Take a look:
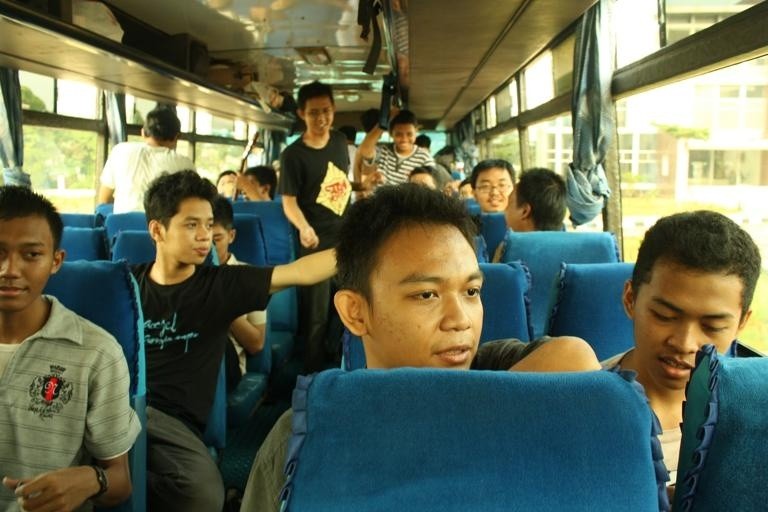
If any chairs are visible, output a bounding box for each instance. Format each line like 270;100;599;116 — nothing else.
39;196;768;512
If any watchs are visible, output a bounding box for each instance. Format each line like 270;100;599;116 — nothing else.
87;462;107;500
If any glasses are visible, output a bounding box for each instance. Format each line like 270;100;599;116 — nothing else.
474;183;513;194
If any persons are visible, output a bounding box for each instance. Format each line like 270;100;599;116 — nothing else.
493;167;568;263
408;134;475;200
130;172;337;511
277;82;384;376
97;110;195;215
2;186;141;511
338;108;382;205
210;195;268;391
471;160;517;227
217;166;277;201
358;96;436;185
603;208;760;499
238;186;600;508
